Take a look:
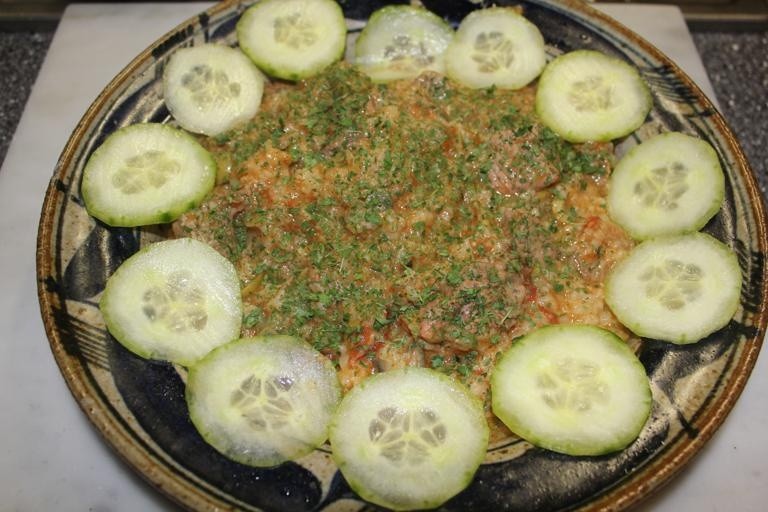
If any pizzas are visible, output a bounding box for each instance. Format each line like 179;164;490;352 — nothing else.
169;59;641;445
